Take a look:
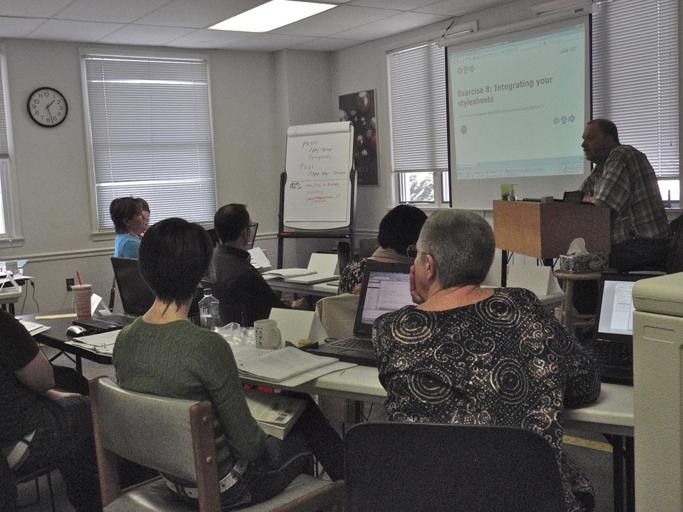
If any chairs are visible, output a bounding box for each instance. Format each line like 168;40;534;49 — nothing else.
110;256;200;321
343;422;592;512
200;279;249;328
1;433;82;511
27;87;69;128
86;375;347;511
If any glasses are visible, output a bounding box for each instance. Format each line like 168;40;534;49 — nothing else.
247;223;257;230
406;244;432;260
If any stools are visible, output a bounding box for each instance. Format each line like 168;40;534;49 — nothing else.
552;269;605;330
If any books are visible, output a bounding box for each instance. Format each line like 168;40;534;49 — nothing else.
17;317;51;338
243;390;305;441
259;267;339;286
229;344;338;382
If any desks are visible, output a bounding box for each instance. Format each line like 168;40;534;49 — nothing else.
479;284;569;315
216;323;634;512
12;308;138;378
492;199;613;289
264;274;343;311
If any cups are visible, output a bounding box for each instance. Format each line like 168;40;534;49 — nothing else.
72;284;92;320
500;183;514;200
254;319;282;350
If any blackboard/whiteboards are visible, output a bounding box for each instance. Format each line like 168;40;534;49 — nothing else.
284;118;354;232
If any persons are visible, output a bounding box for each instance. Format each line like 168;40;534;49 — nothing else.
0;307;104;512
371;208;601;512
338;204;428;298
557;118;669;341
208;203;310;328
109;197;143;260
112;217;346;512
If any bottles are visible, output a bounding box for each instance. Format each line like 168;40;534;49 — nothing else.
197;288;220;330
500;182;517;201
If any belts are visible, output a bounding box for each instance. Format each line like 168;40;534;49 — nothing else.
158;457;250;498
8;427;36;469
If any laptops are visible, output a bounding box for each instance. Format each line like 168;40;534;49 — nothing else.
310;261;416;367
71;313;135;335
580;274;661;387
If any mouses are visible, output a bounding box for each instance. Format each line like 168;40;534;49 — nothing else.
65;325;87;336
